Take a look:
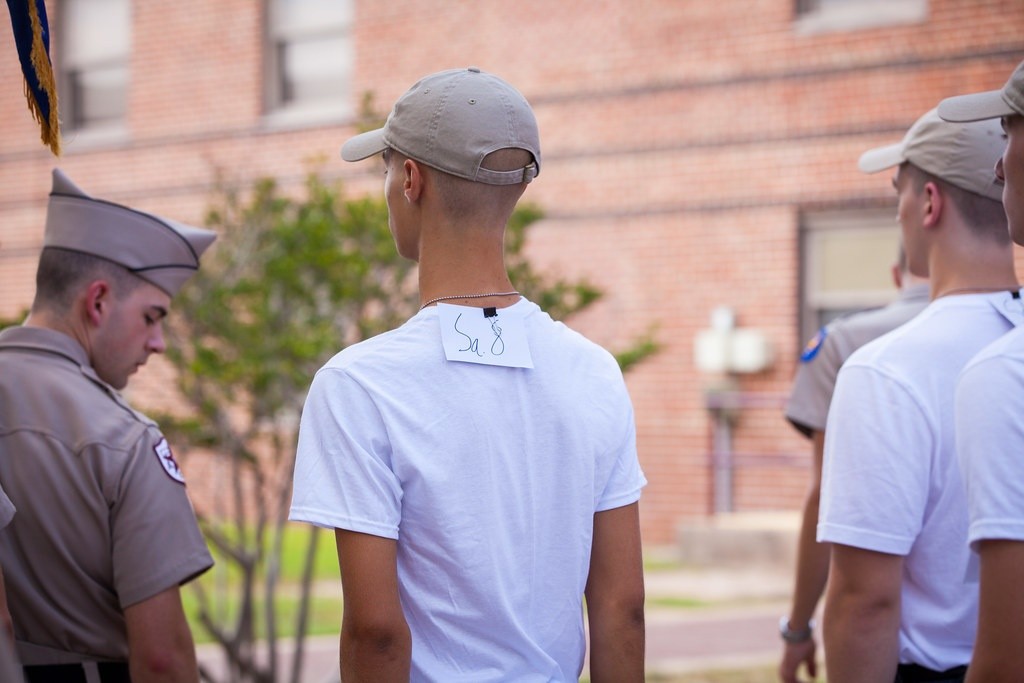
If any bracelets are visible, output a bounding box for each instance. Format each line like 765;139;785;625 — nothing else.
781;621;812;643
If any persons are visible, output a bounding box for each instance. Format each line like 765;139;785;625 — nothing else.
938;60;1024;683
779;241;931;683
816;106;1024;683
0;169;218;683
287;65;650;683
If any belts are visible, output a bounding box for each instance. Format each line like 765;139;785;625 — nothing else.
23;660;131;683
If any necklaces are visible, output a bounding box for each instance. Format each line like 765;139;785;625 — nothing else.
419;291;519;310
930;286;1022;304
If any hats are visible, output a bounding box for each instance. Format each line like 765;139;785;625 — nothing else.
938;63;1024;130
340;67;541;184
42;168;218;301
857;102;1011;203
